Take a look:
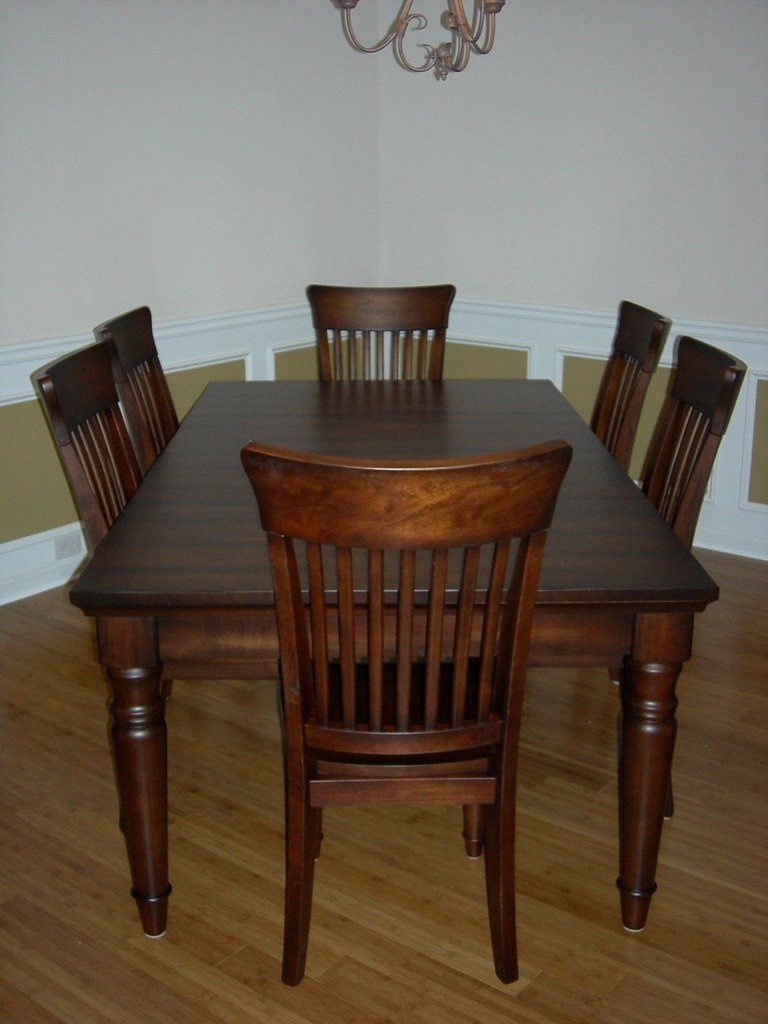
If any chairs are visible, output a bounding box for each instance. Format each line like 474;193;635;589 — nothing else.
588;299;674;472
307;284;457;379
239;439;572;988
93;305;184;477
635;336;749;817
31;339;146;553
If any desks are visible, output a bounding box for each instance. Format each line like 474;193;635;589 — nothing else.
69;380;719;940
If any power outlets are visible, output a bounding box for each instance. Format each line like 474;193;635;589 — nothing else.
55;531;81;560
